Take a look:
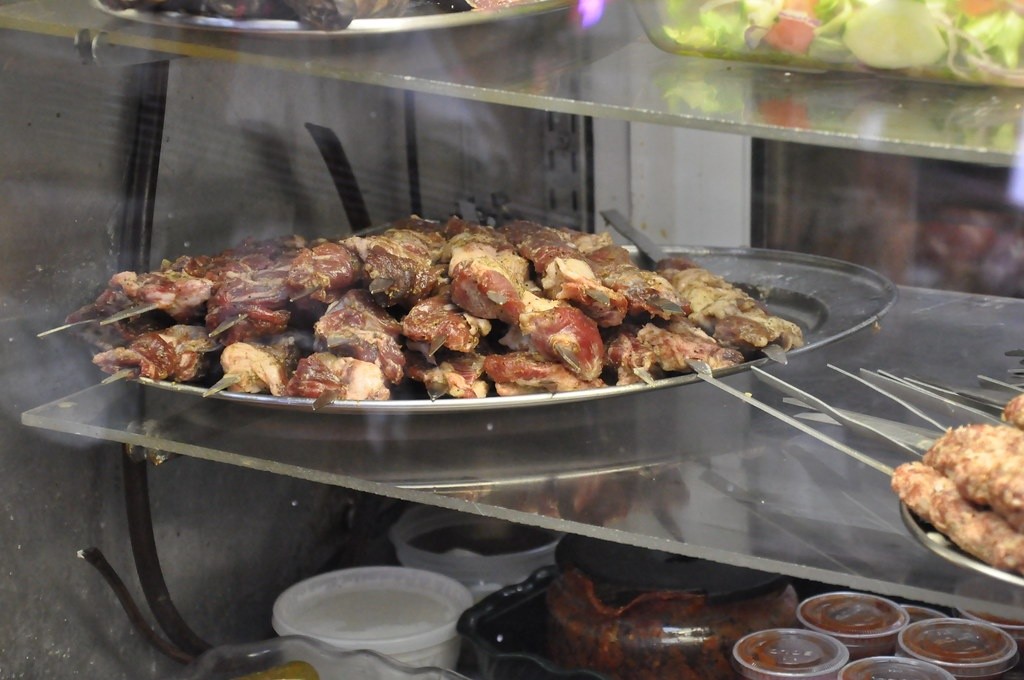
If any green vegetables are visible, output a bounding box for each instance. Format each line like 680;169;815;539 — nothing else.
661;0;1024;75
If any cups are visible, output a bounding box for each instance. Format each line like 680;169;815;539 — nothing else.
269;565;473;680
390;507;562;586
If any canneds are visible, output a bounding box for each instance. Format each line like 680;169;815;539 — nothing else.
731;568;1024;680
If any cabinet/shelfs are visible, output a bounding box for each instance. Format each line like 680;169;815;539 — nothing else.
0;6;1024;680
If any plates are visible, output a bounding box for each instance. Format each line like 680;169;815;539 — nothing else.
64;244;901;417
175;634;477;680
900;500;1024;588
93;0;600;43
